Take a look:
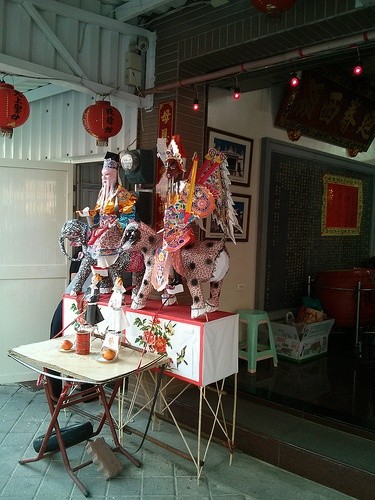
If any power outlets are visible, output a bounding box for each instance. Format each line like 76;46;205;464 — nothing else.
237;282;246;291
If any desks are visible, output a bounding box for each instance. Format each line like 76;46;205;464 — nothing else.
7;331;171;498
59;292;240;488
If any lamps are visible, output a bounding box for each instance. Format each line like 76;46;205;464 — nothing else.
192;85;199;110
352;46;363;75
288;61;299;88
233;76;240;100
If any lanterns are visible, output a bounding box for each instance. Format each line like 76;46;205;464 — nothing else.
0;80;30;139
82;101;123;147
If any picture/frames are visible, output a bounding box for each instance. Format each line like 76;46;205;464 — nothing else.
205;126;254;188
201;192;252;242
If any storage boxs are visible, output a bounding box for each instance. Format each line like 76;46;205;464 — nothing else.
269;318;336;361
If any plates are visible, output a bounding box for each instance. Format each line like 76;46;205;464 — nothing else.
94;355;117;362
56;345;75;352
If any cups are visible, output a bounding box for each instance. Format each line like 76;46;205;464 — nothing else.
76;331;90;354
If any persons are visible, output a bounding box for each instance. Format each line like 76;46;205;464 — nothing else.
83;273;105;325
163;139;243;294
70;247;83;282
83;151;138;277
105;277;131;332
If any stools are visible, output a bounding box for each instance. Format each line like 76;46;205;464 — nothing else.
234;308;278;373
